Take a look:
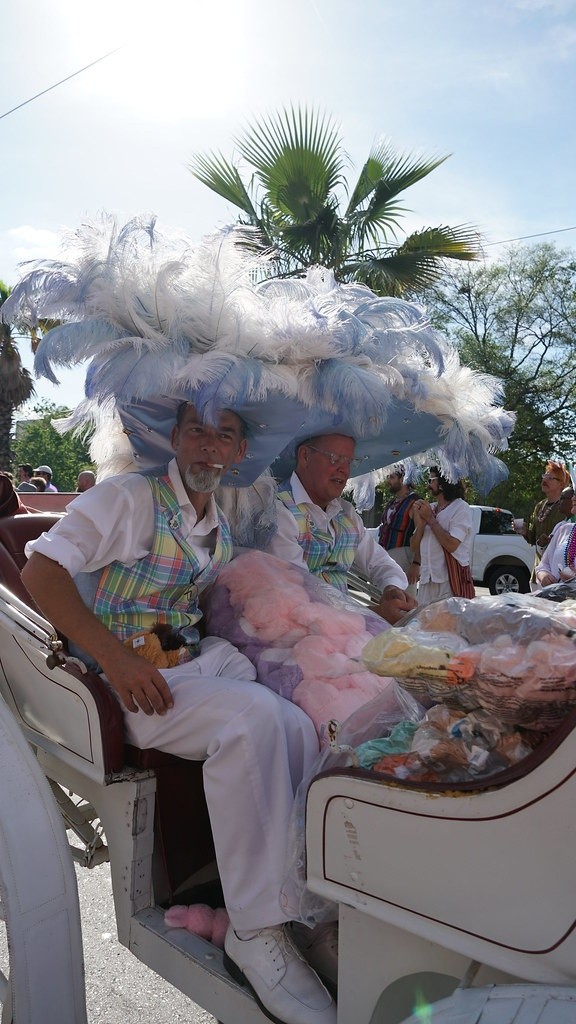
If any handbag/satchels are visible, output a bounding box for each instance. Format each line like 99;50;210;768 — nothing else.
444;549;475;598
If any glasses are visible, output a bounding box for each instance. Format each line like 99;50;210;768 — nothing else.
428;477;440;483
542;475;561;483
307;445;361;470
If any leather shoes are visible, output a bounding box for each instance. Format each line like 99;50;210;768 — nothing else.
222;923;337;1024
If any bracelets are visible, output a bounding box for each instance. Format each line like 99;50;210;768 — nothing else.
413;561;421;566
427;515;439;529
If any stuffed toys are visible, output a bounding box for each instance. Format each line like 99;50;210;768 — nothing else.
204;550;576;784
123;623;200;668
164;904;227;946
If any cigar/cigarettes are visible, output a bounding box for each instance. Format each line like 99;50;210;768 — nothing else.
208;463;225;468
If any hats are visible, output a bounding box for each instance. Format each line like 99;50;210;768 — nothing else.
15;482;37;492
33;465;52;476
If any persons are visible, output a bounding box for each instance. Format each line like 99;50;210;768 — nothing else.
536;487;574;556
17;464;34;483
410;466;474;605
535;492;576;587
78;471;96;492
19;402;339;1024
32;466;57;492
29;477;46;492
266;433;420;626
512;459;571;593
378;464;425;598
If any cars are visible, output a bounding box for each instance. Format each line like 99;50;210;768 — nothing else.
365;503;538;597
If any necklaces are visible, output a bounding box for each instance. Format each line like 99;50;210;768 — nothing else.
438;503;449;512
564;523;576;574
538;498;558;523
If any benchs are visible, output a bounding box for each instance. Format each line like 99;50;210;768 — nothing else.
1;513;225;912
306;717;576;1024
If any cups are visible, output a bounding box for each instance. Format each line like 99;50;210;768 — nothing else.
513;518;524;533
